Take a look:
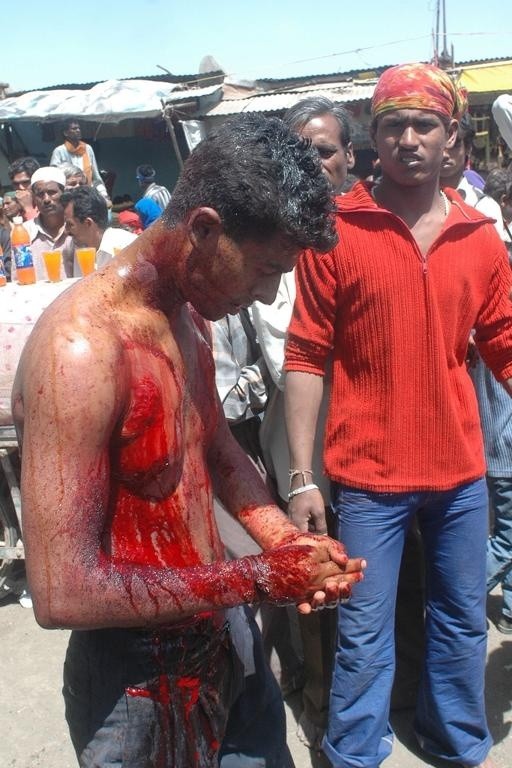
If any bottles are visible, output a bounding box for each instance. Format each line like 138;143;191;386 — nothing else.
11;216;37;285
0;242;7;287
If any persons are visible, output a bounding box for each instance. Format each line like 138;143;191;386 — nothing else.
0;117;172;282
204;62;512;768
12;113;366;768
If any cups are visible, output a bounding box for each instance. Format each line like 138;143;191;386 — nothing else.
44;251;61;283
76;247;96;278
